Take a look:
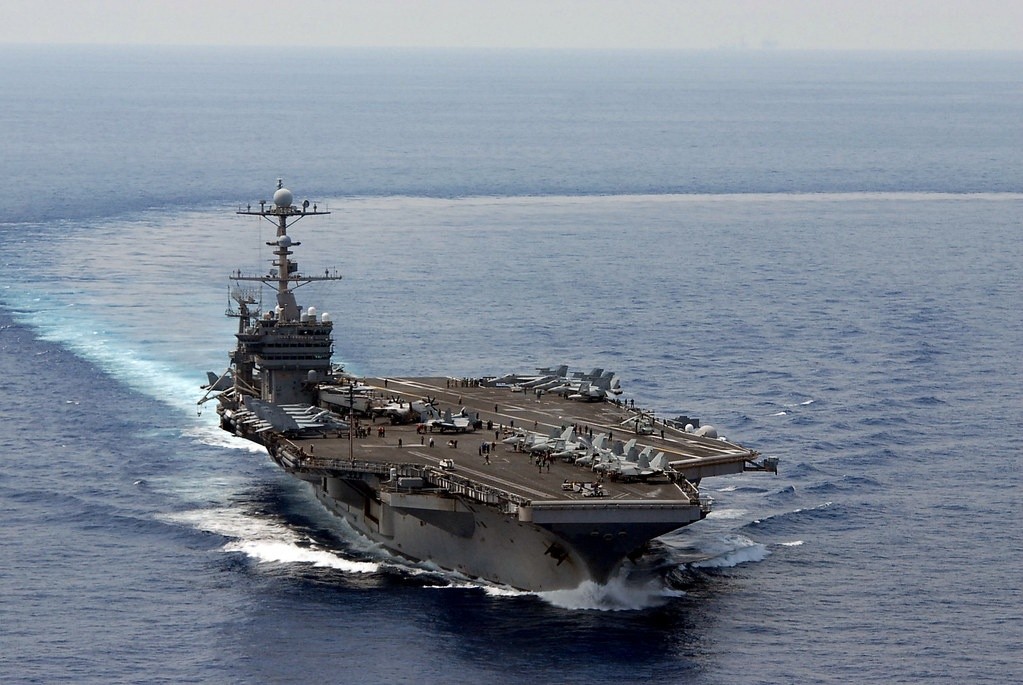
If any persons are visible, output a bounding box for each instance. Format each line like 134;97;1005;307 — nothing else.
520;382;573;400
510;419;555;473
310;444;313;453
339;409;387;441
561;466;621;494
557;419;617;441
639;412;667;440
445;377;482;388
397;396;509;468
384;379;389;388
610;397;637;409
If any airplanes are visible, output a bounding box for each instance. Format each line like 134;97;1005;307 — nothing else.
486;363;624;404
425;406;482;433
500;425;670;482
232;395;351;440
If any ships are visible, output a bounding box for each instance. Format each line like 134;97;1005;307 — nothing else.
196;175;781;608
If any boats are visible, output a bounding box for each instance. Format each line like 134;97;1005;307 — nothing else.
315;378;375;415
